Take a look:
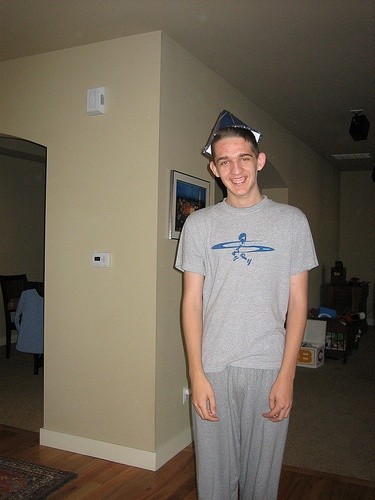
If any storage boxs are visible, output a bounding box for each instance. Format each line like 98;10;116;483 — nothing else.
293;365;309;391
296;319;328;368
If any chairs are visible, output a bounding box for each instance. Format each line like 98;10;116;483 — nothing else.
0;274;45;376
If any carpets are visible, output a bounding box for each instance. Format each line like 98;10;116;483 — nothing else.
0;455;79;500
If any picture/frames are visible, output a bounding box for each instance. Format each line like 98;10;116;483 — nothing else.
169;169;210;240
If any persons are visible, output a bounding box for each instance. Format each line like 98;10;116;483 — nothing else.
174;127;319;500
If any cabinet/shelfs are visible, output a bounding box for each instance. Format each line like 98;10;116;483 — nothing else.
320;284;369;316
